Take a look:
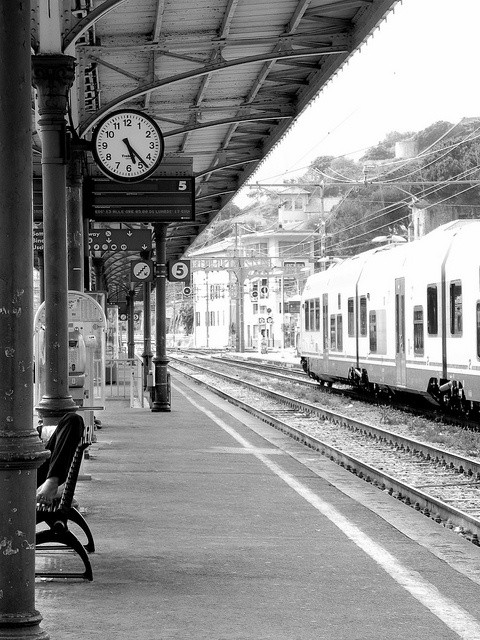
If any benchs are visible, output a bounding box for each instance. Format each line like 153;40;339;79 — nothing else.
35;425;95;581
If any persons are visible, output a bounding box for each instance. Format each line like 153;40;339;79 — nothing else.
36;412;84;505
94;416;102;431
36;420;43;438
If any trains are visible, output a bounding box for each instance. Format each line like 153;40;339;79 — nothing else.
300;219;480;427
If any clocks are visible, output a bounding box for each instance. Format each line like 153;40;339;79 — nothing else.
90;108;165;185
